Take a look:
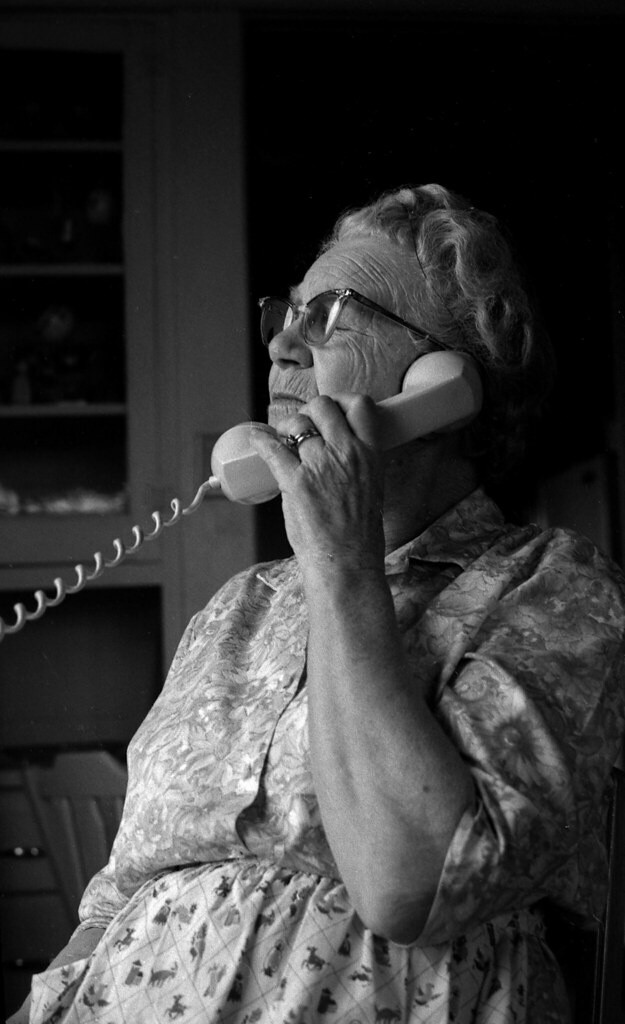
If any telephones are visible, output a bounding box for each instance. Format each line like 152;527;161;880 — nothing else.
211;349;483;509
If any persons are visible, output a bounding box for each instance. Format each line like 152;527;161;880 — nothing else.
0;181;625;1024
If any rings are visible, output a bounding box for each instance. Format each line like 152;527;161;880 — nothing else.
279;427;318;453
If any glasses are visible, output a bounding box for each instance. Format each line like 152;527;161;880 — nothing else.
258;289;448;351
